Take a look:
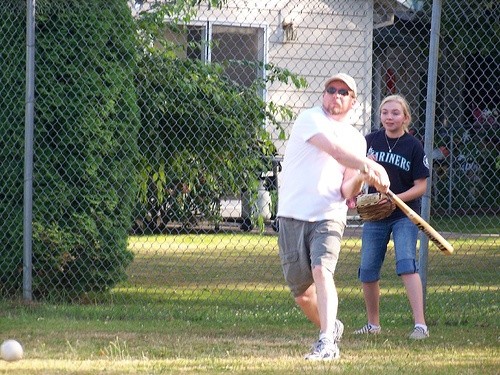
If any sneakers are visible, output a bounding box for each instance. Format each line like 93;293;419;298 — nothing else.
408;327;430;341
303;333;340;362
349;323;382;335
334;319;345;343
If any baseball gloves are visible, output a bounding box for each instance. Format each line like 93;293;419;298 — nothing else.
355;191;397;222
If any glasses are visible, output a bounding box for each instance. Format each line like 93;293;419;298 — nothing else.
326;87;350;96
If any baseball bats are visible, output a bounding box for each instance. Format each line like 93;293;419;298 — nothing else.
359;166;455;257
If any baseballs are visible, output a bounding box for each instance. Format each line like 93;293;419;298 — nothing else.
1;339;25;363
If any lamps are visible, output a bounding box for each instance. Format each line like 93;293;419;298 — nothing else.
281;16;298;42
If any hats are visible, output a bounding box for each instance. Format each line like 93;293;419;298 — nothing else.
325;72;357;98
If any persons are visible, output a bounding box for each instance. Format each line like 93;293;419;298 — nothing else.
433;145;448;159
350;94;431;341
277;73;391;359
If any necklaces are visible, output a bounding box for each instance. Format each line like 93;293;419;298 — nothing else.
384;132;400;152
